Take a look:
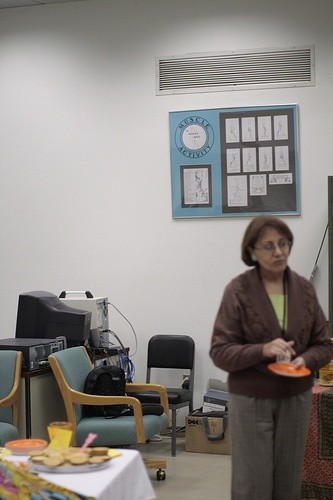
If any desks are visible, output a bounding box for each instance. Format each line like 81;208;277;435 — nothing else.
20;347;130;439
2;447;156;500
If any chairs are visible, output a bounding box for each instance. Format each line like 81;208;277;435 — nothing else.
0;350;22;448
127;334;195;457
48;345;169;481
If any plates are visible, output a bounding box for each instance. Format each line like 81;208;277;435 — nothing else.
268;363;311;378
27;456;113;473
5;439;46;455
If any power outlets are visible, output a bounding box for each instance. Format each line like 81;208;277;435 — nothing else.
183;375;190;382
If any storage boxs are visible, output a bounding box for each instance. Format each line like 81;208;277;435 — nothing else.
202;378;228;413
185;416;231;455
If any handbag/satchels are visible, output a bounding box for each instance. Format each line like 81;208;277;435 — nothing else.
83;365;164;419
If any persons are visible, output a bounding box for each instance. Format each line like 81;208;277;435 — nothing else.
209;217;333;500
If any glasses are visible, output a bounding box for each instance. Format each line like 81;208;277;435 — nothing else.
254;240;291;252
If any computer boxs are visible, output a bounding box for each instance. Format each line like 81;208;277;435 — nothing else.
0;338;65;371
58;297;110;349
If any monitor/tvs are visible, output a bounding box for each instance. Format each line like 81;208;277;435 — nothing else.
15;291;92;349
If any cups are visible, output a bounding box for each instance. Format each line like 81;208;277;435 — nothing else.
49;422;73;448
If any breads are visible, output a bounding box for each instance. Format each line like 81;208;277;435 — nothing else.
29;447;110;466
